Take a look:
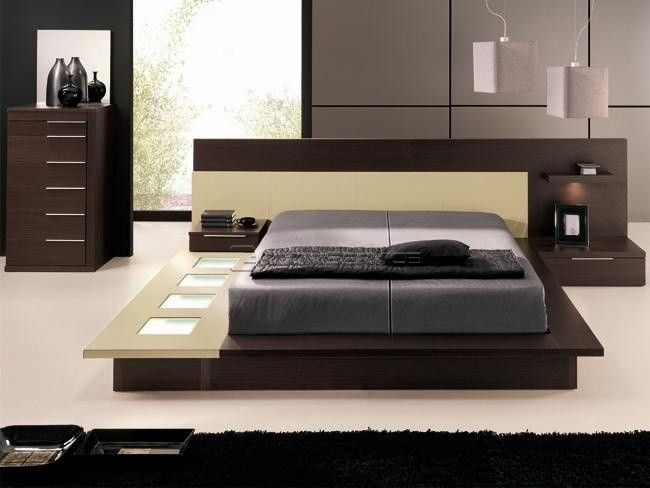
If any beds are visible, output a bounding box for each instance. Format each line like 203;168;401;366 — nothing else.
210;206;605;363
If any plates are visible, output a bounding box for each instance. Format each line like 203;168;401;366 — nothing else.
237;222;258;227
75;427;197;465
0;423;85;475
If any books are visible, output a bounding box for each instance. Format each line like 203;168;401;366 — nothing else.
200;210;236;227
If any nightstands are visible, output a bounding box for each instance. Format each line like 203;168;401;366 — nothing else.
188;218;271;252
532;235;646;287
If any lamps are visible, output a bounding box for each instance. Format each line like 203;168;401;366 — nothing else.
544;0;610;121
469;0;537;94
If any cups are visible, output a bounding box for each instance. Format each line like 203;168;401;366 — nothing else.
236;217;256;225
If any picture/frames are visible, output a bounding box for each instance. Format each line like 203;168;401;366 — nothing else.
553;201;591;248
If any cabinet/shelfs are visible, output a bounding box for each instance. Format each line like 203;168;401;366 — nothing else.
4;104;113;272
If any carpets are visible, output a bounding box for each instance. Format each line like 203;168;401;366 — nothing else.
0;425;648;485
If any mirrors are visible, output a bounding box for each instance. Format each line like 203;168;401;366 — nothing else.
37;28;111;105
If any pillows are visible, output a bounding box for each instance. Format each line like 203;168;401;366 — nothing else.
380;237;473;266
